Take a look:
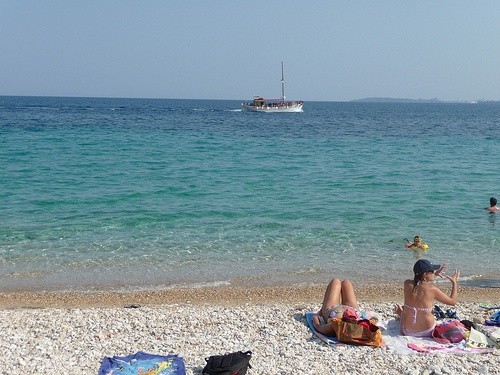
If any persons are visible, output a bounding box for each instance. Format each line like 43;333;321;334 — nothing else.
407;235;430;250
401;258;460;336
484;196;500;212
312;279;380;342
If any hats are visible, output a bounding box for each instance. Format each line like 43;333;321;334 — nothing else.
343;309;359;322
413;259;441;273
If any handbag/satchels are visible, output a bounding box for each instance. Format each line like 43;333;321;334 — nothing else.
436;320;465;343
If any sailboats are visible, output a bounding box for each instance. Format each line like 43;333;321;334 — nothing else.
241;61;305;113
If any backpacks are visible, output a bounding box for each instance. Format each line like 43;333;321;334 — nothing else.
202;350;252;375
